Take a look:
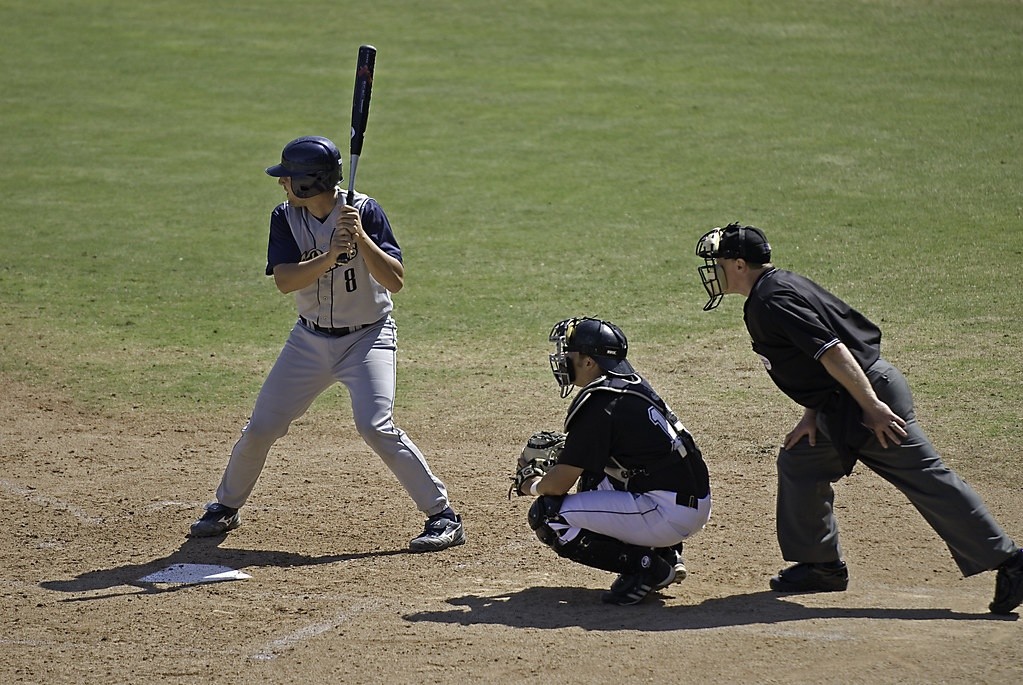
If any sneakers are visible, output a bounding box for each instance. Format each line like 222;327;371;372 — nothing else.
409;514;466;551
769;559;849;593
989;548;1023;613
665;550;687;582
602;554;675;607
189;500;240;536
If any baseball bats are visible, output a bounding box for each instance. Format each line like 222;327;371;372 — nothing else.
335;44;377;265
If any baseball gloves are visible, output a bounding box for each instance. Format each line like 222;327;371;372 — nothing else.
507;429;569;501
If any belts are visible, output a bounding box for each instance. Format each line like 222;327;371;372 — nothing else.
299;316;369;337
675;493;699;510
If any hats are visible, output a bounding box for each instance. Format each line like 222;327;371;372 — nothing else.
698;221;773;261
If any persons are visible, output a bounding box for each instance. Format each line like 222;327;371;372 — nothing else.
189;135;466;551
696;223;1023;615
516;315;711;607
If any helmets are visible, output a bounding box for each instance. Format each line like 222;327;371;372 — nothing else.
558;317;636;376
265;135;344;199
